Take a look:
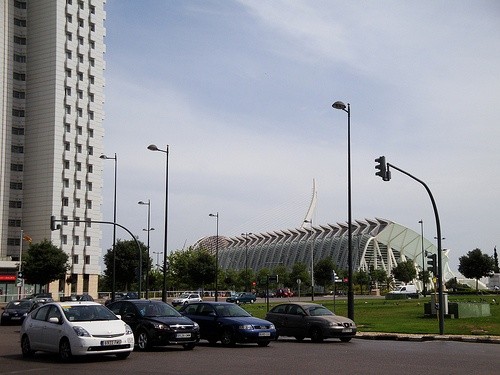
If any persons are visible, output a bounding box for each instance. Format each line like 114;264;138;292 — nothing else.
287;286;291;297
251;288;256;295
279;288;284;298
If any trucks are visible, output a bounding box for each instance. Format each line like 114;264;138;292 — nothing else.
389;285;416;294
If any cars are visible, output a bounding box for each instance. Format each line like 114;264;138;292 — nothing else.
227;292;257;305
102;301;200;353
260;303;357;342
177;302;279;345
0;290;138;325
21;301;136;361
171;292;201;307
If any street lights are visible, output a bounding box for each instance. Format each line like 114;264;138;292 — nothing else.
153;251;164;302
139;200;151;300
146;141;169;303
143;226;156;301
99;152;117;300
419;219;428;297
208;212;219;301
330;100;354;331
241;232;252;292
304;218;315;301
434;237;447;292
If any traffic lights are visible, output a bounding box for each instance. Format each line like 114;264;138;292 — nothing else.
375;155;386;179
427;253;436;275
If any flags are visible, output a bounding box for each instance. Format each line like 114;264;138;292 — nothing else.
22;233;32;243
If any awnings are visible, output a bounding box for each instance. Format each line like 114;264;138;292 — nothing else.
0;274;16;280
